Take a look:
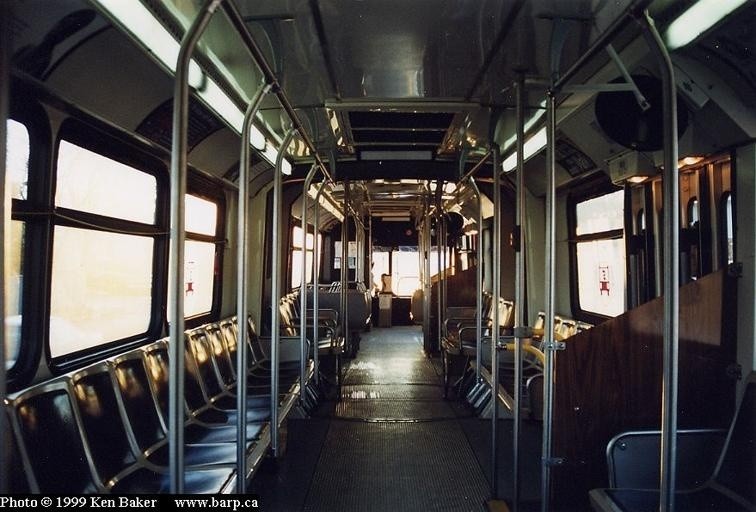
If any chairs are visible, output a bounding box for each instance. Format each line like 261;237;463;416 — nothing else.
588;370;755;512
440;290;595;416
